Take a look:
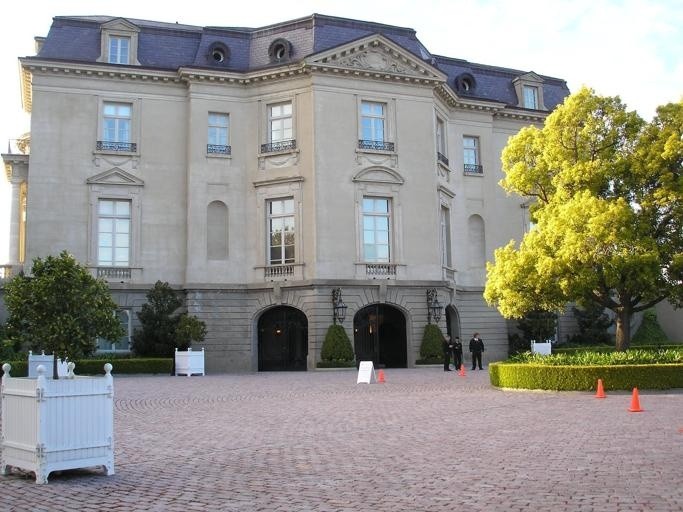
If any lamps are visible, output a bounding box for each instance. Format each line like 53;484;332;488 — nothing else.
331;287;348;324
426;287;443;324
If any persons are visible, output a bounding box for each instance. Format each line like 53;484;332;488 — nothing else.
442;335;454;371
452;336;463;371
469;333;484;370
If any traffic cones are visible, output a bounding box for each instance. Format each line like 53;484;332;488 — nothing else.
379;369;384;382
626;386;646;412
593;378;608;398
458;363;466;376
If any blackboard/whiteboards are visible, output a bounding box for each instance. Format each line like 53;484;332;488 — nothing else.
357;360;375;383
